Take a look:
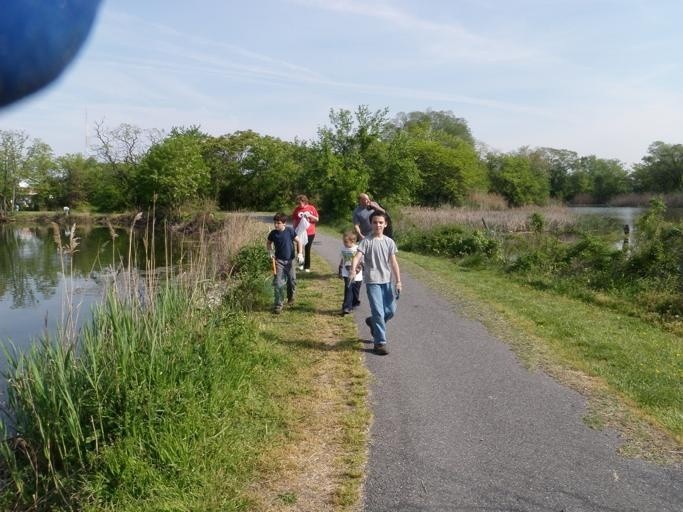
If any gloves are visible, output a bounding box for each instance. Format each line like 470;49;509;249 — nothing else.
393;283;401;299
269;250;274;259
348;269;356;287
296;253;304;262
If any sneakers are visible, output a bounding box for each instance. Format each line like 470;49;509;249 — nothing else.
299;265;303;270
375;344;388;354
275;304;283;314
343;308;351;314
305;268;310;273
366;318;375;336
288;298;295;304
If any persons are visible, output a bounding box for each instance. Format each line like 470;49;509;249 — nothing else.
266;213;303;314
352;193;386;245
345;210;404;357
291;193;320;274
335;230;365;318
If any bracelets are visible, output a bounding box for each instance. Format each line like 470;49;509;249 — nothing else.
338;263;342;268
396;279;401;282
